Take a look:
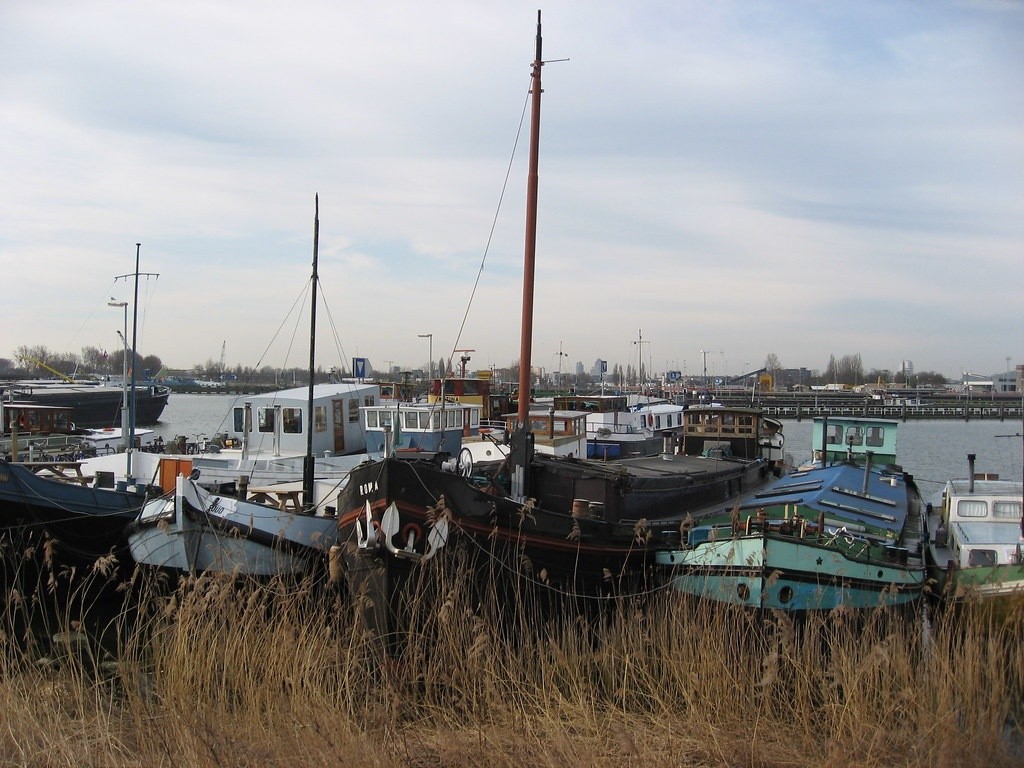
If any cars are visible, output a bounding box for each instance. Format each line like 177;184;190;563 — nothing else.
787;384;812;392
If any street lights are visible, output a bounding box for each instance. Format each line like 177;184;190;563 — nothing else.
815;376;818;395
418;333;432;380
488;364;495;388
700;350;709;387
108;301;131;447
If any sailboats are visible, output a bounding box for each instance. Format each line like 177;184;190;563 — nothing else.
332;9;794;674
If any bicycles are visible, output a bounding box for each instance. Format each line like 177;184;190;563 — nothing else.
0;438;54;463
55;432;239;465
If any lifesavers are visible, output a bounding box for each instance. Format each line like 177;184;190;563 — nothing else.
648;412;653;426
20;415;28;425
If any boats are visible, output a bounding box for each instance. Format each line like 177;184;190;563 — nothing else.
923;453;1024;611
656;410;928;638
1;375;172;430
128;188;508;627
0;381;686;659
